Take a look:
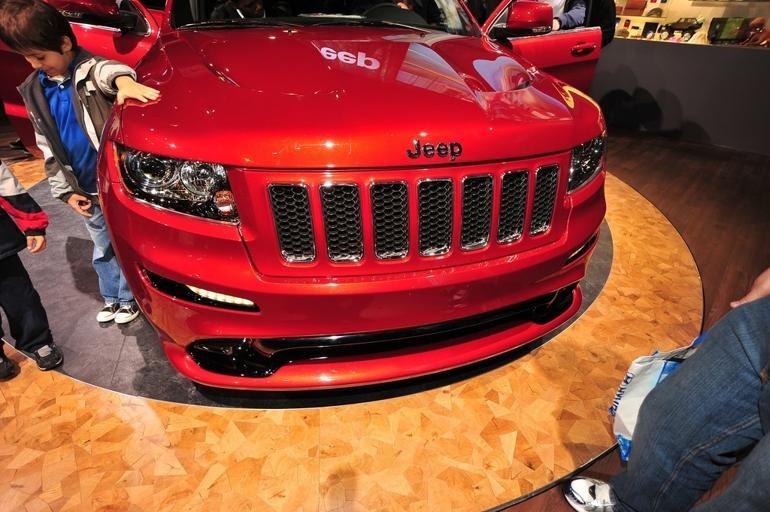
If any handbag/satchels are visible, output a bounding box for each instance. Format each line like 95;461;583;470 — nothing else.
609;336;704;462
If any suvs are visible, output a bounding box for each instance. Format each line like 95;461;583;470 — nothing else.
0;0;608;391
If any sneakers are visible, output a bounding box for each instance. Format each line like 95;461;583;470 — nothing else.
32;342;63;370
563;476;614;512
0;353;19;379
96;299;140;324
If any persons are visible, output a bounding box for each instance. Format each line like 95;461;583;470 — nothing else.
0;161;64;379
0;1;160;326
394;0;412;11
537;0;616;47
210;0;262;19
561;266;770;512
